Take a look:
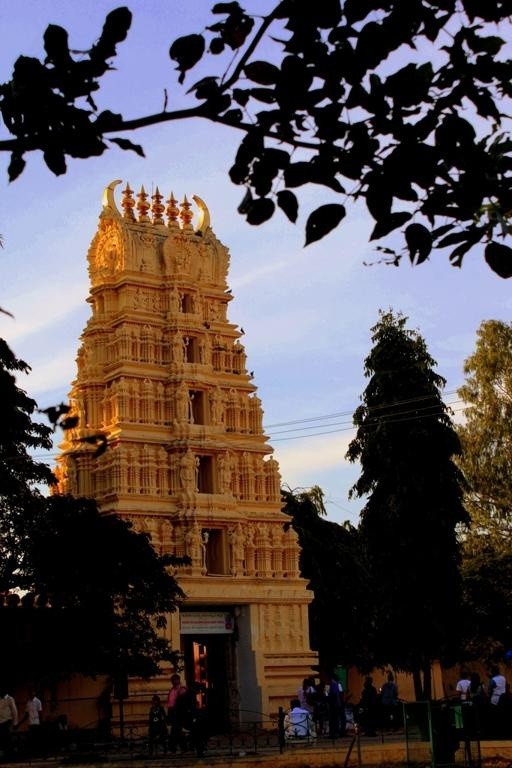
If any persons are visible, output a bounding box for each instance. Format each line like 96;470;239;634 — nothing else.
22;685;48;727
285;669;400;743
168;672;190;714
146;691;169;729
455;668;472;701
0;682;19;764
486;663;511;707
466;673;490;703
